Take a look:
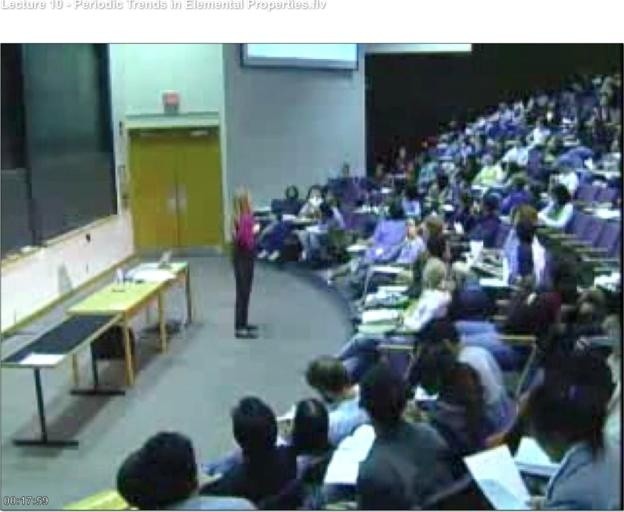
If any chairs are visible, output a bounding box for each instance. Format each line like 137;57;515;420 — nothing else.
255;90;624;511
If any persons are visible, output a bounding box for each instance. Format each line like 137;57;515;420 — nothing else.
115;69;620;510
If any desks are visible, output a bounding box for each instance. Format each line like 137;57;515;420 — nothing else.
0;262;190;448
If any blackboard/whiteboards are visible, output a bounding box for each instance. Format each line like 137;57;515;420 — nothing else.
0;43;122;268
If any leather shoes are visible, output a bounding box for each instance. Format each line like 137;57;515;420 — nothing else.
235;325;259;339
